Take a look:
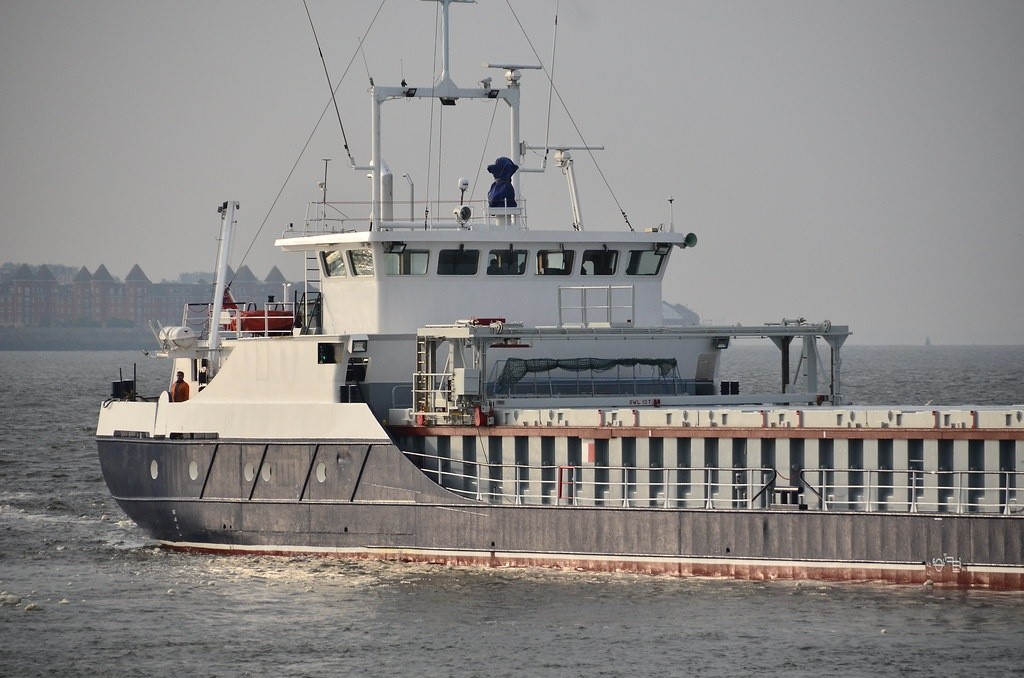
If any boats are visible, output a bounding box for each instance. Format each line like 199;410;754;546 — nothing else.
96;0;1024;597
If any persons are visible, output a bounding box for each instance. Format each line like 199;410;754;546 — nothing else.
171;371;189;402
581;255;587;275
487;259;525;274
